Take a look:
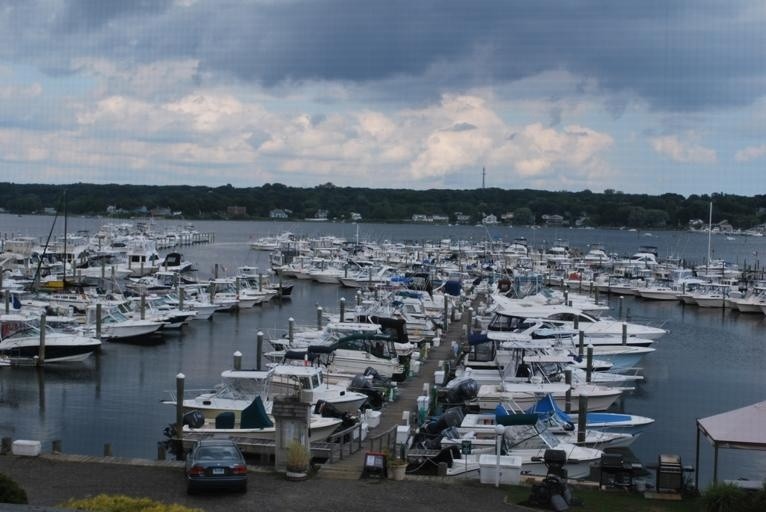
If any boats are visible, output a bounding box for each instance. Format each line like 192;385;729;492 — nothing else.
0;218;766;482
0;322;101;369
183;413;342;456
60;305;168;340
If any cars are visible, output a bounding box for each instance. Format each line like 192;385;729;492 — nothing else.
184;439;248;496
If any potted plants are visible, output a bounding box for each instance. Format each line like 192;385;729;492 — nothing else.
278;438;310;478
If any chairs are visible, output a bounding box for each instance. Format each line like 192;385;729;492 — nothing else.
603;446;685;493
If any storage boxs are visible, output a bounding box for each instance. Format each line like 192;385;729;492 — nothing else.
12;440;42;458
